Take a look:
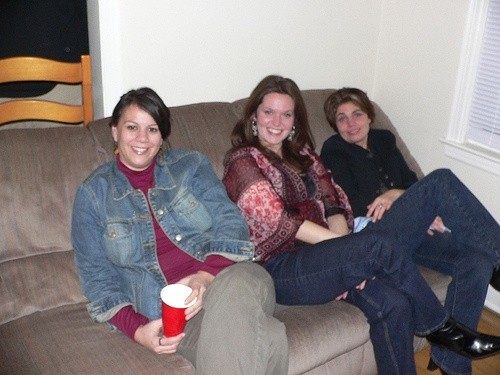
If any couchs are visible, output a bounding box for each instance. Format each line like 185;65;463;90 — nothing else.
0;89;452;375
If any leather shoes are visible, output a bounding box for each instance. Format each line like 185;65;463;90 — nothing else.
426;318;500;360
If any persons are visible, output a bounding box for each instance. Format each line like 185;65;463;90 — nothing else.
319;88;500;375
70;88;288;375
222;76;500;375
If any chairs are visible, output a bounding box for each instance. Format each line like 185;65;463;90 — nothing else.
0;55;94;128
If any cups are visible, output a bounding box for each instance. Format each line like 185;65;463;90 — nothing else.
160;284;198;339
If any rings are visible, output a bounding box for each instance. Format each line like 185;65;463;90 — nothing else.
376;204;383;208
159;338;164;346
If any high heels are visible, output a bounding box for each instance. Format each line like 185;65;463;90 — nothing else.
427;357;447;375
490;263;500;291
